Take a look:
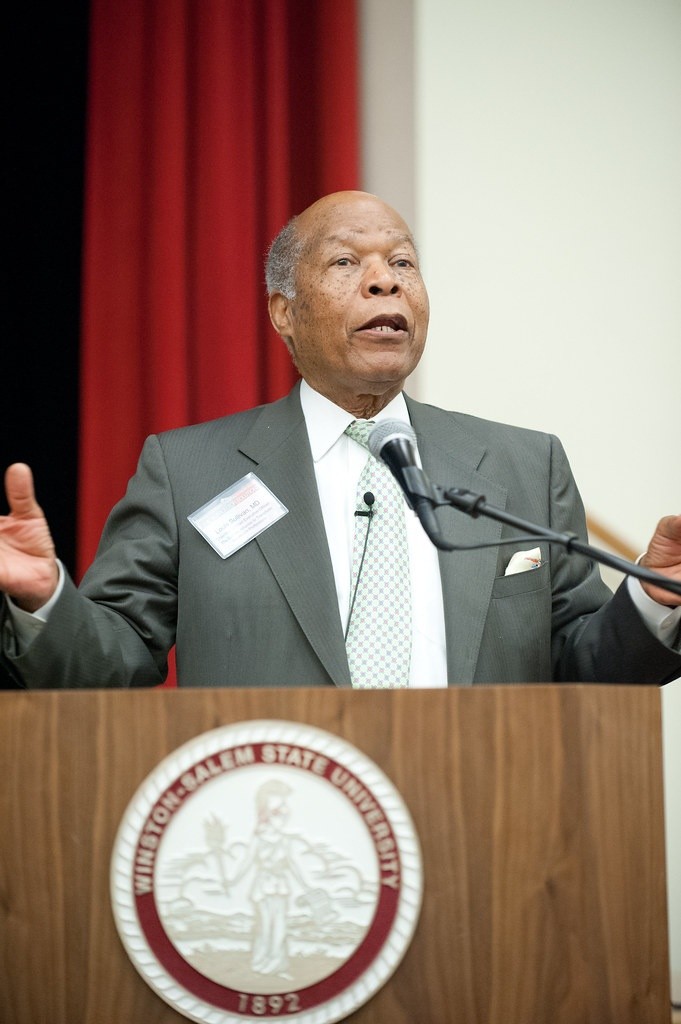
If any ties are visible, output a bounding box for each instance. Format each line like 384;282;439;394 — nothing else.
342;418;413;690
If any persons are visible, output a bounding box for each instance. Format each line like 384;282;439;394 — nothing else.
0;186;680;685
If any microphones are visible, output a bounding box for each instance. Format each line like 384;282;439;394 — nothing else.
355;492;374;517
368;419;452;552
